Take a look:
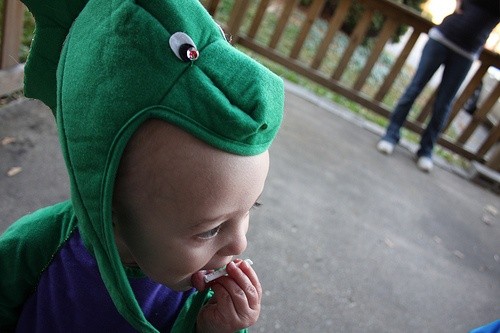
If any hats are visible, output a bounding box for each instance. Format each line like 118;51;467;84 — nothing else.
22;0;284;333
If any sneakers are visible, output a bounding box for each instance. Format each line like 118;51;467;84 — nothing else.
378;140;394;155
417;157;433;170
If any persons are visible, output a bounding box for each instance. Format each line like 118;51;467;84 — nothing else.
378;0;500;170
0;1;284;333
